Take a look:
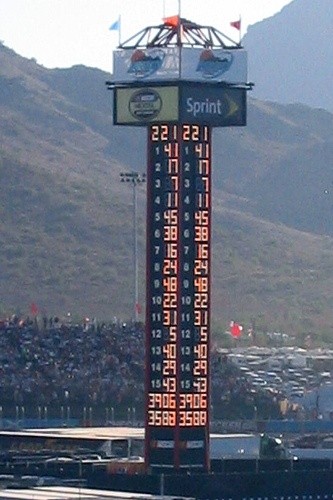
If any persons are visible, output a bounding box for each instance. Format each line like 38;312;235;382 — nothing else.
0;314;285;420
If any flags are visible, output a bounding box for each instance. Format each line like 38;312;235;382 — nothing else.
109;15;240;31
136;302;141;313
32;303;37;311
